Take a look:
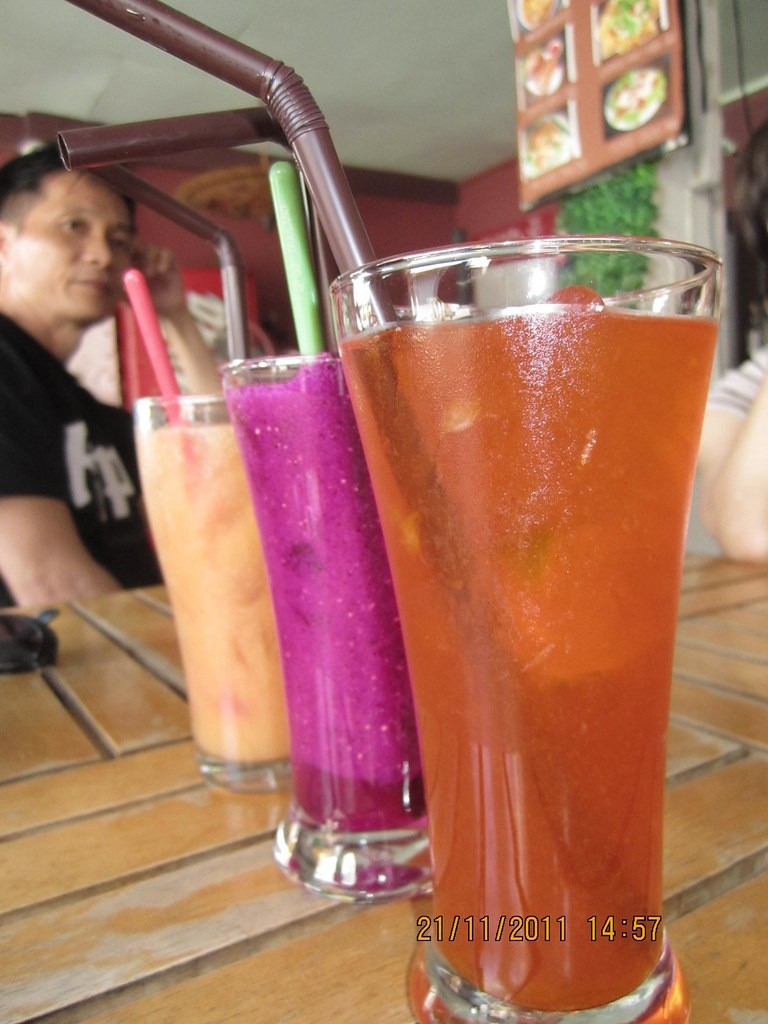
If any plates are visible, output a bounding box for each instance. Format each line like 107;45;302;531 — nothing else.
516;0;559;31
604;69;664;130
526;54;561;95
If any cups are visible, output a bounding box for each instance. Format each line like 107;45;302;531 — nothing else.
330;230;722;1024
223;360;429;899
131;396;296;793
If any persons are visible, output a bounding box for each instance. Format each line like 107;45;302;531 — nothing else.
694;120;768;567
0;141;229;607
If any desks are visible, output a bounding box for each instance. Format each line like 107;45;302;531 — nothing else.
0;544;767;1023
